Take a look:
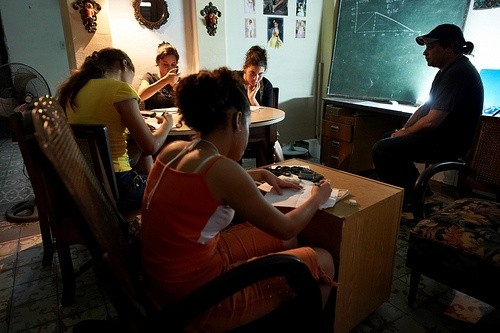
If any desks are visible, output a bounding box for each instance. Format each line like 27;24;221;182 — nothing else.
244;156;405;333
319;94;500;192
139;105;288;143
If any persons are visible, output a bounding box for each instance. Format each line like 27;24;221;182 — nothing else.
14;92;35;114
269;18;283;42
55;42;285;219
372;23;484;205
138;67;335;333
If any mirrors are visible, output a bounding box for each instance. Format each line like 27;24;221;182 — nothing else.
132;0;170;30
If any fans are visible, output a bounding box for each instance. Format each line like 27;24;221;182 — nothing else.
0;63;51;226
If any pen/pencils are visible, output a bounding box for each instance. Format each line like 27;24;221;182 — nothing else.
161;111;166;117
315;182;321;186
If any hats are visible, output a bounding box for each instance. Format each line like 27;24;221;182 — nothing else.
416;23;464;46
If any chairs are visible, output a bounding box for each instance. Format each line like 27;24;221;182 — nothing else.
405;116;500;311
12;94;323;333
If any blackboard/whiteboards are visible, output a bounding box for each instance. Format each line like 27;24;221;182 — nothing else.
327;0;473;113
